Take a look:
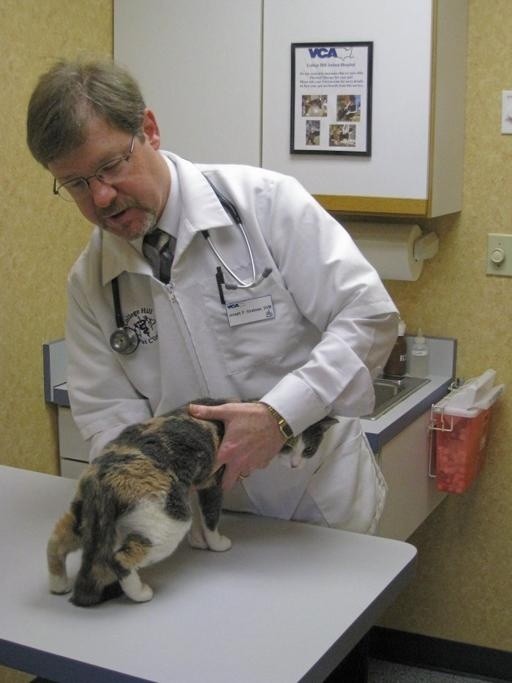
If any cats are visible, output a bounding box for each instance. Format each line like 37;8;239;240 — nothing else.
44;393;340;608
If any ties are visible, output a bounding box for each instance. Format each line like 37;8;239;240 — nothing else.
141;228;172;284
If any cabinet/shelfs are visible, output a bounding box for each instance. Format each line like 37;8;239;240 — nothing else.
110;0;466;220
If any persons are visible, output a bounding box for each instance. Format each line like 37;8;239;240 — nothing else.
24;51;400;682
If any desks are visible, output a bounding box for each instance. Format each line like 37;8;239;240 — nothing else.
2;462;417;681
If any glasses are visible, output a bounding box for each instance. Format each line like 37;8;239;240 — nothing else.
52;134;137;201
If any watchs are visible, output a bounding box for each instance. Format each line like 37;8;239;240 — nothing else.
251;398;297;447
